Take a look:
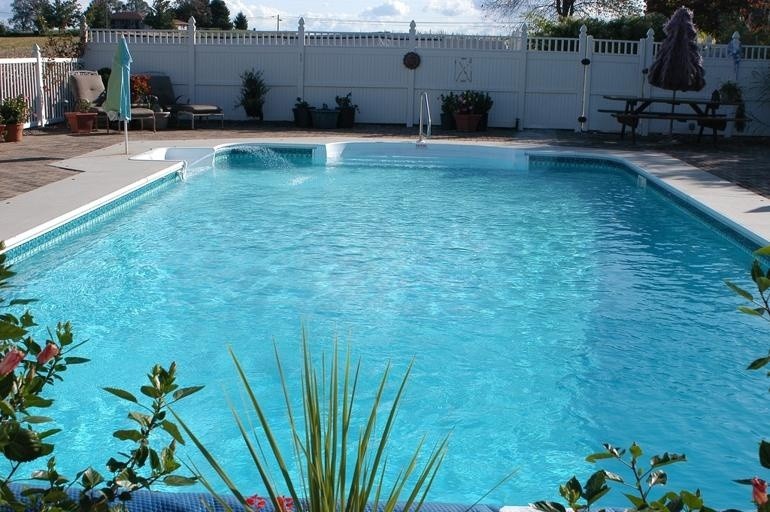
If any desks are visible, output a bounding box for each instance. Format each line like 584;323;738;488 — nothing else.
603;93;745;145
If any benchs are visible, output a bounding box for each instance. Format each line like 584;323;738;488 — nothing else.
597;109;728;119
610;113;753;121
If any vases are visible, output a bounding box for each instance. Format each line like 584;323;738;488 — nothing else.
64;111;80;133
0;124;6;142
143;111;171;130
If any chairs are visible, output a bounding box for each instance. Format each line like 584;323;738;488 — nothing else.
69;70;157;135
149;75;224;129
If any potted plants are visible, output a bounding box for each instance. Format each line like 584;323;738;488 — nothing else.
292;91;362;128
0;93;34;142
718;76;743;102
439;90;494;133
75;98;98;134
232;66;271;119
711;89;720;101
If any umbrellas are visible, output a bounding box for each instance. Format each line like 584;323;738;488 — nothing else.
647;6;706;140
101;31;133;154
